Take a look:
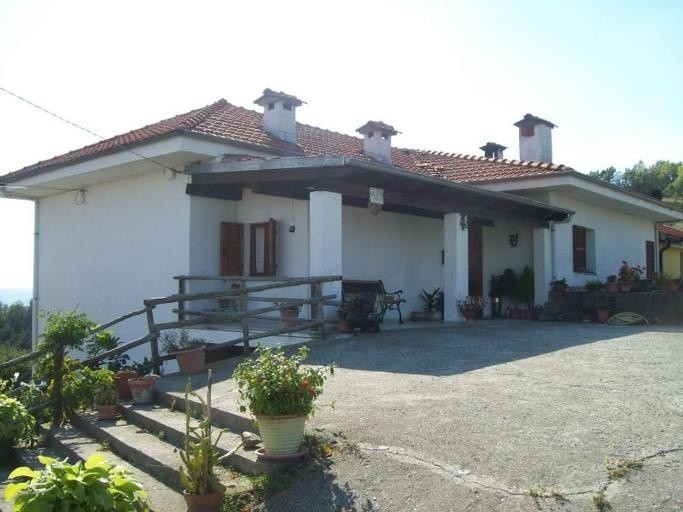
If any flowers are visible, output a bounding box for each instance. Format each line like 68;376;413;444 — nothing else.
455;295;489;307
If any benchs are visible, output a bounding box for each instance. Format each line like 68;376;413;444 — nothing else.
341;276;408;325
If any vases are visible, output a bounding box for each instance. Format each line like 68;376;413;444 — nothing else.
410;309;423;322
463;308;476;319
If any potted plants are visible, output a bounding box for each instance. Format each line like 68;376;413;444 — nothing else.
488;260;683;324
416;283;442;323
